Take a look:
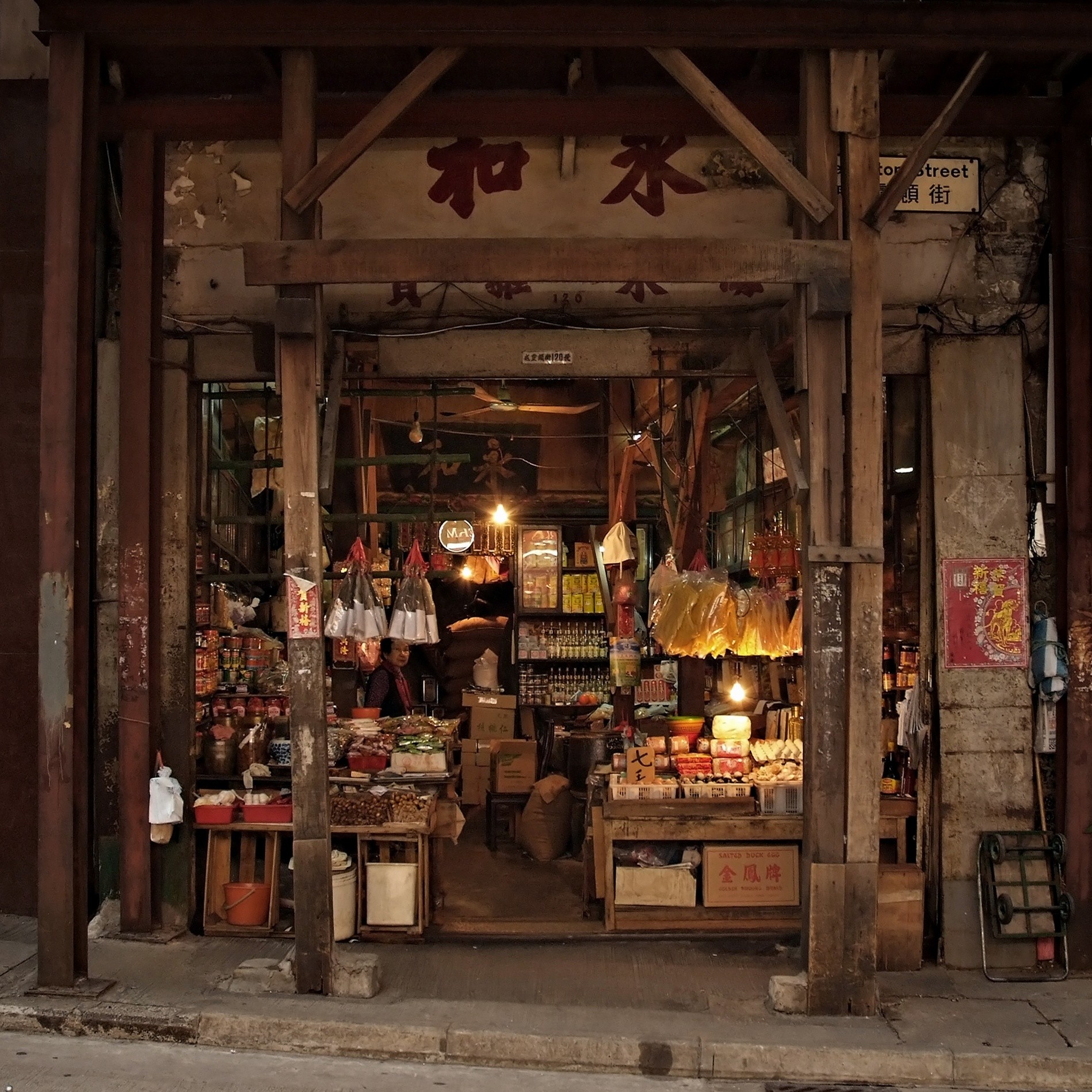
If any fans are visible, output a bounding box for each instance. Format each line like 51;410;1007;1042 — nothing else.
448;379;600;418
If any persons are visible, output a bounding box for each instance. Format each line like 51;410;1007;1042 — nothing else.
363;636;414;719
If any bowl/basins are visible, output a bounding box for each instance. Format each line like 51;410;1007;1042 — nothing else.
348;756;387;772
671;731;701;748
352;708;381;720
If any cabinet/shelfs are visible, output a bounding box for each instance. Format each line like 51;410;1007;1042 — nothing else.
511;517;705;716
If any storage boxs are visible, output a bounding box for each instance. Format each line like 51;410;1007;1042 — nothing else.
526;541;605;613
346;756;389;769
702;841;800;908
390;752;447;772
460;694;537;805
634;665;677;704
194;800;239;824
613;867;697;907
240;802;293;823
609;774;803;813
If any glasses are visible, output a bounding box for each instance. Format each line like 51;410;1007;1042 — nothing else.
392;647;412;654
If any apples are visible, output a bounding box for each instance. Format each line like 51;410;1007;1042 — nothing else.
576;693;598;706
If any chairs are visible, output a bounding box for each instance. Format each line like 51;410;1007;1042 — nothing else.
486;720;554;852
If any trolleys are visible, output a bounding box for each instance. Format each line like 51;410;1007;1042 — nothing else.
976;830;1076;982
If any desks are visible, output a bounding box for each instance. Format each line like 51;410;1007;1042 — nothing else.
193;819;434;914
603;815;912;933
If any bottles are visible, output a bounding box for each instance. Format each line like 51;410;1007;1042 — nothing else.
640;628;655;658
518;618;612;705
881;640;919;796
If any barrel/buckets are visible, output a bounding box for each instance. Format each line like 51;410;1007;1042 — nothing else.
222;883;271;926
332;867;355;941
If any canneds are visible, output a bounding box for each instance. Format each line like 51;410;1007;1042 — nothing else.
195;525;245;721
518;663;549;705
897;644;919;688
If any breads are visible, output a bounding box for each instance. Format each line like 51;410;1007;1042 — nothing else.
750;738;805;782
